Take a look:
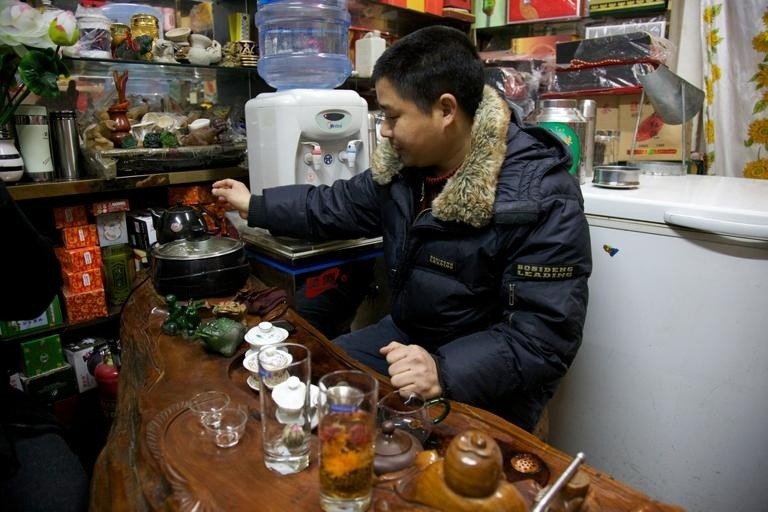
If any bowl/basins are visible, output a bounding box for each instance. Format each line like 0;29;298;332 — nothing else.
186;390;231;426
200;406;248;449
592;164;643;190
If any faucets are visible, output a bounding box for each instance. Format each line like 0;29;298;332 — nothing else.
300;140;320;172
338;139;362;169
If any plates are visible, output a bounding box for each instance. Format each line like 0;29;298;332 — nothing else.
275;407;323;431
246;370;291;392
244;346;290;359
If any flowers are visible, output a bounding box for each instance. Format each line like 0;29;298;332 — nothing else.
0;1;82;132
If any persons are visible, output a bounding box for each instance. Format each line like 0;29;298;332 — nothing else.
211;26;592;433
0;179;91;512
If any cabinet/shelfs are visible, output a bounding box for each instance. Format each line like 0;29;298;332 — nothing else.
0;1;472;345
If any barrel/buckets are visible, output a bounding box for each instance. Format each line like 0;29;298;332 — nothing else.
355;29;387;79
255;0;353;91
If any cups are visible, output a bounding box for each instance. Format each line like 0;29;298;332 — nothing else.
270;376;320;424
242;344;294;387
243;321;290;354
256;342;312;476
317;368;381;511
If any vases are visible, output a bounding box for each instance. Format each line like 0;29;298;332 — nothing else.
0;138;25;186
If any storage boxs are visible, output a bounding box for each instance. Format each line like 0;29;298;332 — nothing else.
566;93;694;165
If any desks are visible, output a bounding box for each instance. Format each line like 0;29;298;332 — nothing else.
88;268;688;510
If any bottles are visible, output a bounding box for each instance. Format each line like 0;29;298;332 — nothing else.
13;105;55;182
50;110;82;181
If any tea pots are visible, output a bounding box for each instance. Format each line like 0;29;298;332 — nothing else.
144;201;223;247
376;388;453;443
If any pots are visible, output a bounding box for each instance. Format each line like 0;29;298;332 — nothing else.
146;228;252;303
